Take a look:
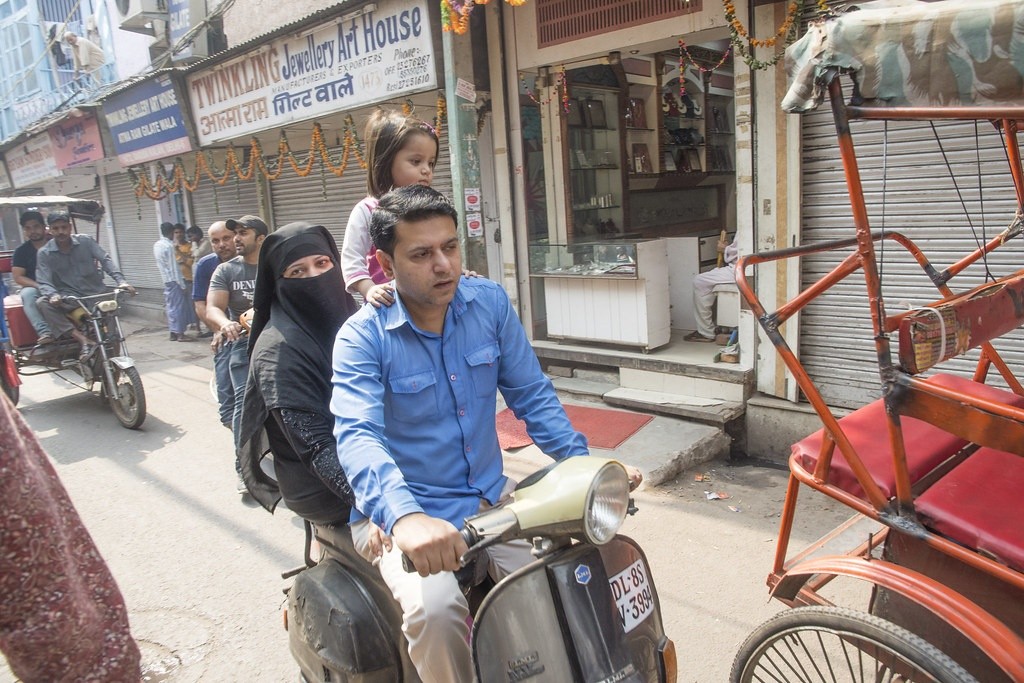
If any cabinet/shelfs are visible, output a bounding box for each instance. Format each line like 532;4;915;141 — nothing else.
570;126;619;212
663;114;707;175
626;127;659;178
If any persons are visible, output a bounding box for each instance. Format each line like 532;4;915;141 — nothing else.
191;215;269;494
329;183;642;683
340;108;485;309
34;209;137;364
64;31;105;89
10;212;57;344
151;221;213;341
237;221;393;557
682;229;738;343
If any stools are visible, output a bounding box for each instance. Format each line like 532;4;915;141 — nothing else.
908;404;1024;582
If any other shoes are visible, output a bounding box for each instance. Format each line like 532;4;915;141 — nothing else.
37;333;54;345
170;332;177;341
237;479;248;494
196;332;213;338
178;333;192;342
684;331;713;343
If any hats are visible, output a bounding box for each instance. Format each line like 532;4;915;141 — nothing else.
47;211;70;225
226;215;268;237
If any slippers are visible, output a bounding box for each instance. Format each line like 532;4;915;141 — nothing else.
79;343;99;364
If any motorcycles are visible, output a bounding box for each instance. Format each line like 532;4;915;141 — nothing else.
211;305;255;349
282;452;684;683
0;195;147;431
732;1;1024;682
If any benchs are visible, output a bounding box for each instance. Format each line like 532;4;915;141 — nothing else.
0;256;40;348
